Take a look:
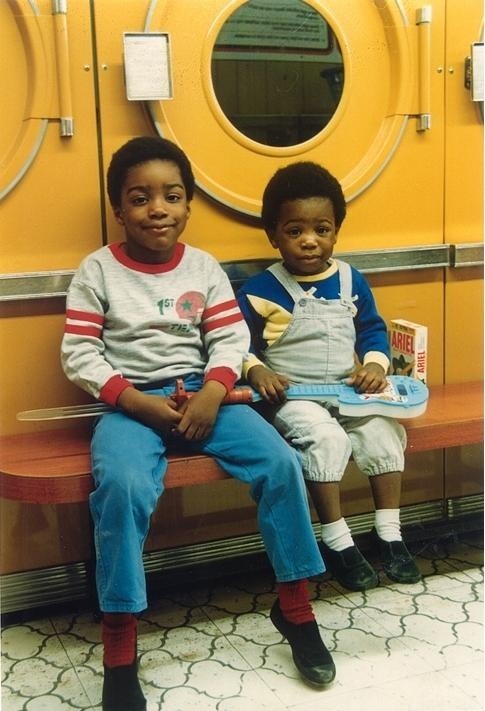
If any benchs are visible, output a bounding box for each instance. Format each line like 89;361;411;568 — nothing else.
0;378;484;507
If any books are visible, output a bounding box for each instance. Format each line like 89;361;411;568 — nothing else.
387;316;429;385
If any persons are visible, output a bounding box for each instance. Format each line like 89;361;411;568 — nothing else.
57;134;338;711
235;159;422;592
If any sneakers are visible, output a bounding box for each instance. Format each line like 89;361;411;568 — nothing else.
321;539;379;592
101;628;146;711
270;599;336;688
372;527;422;585
